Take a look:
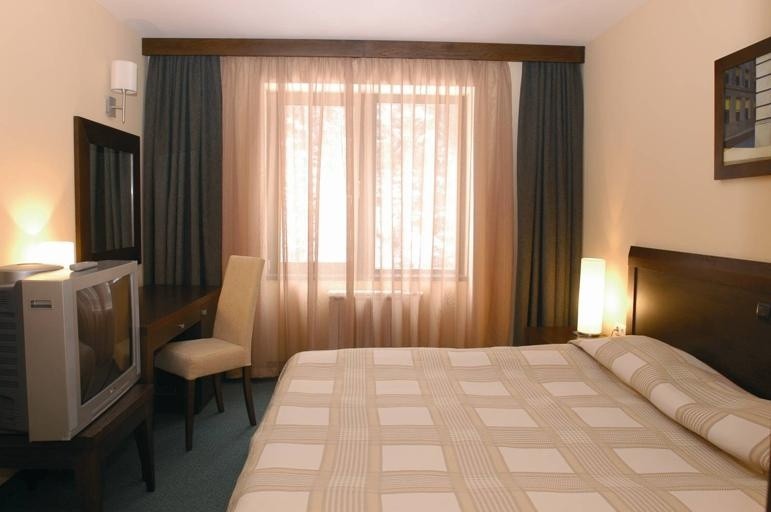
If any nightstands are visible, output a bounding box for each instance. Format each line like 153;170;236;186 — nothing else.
524;325;609;345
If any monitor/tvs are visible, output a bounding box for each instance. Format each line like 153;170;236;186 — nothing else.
0;260;142;443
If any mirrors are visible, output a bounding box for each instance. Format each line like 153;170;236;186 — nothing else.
73;115;142;265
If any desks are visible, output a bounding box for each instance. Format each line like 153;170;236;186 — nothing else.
138;286;222;492
0;384;155;511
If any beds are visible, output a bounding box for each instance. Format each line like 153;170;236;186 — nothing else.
223;246;771;512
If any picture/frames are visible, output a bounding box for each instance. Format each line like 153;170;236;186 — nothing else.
714;37;771;180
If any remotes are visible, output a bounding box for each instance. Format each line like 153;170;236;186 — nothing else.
70;261;97;272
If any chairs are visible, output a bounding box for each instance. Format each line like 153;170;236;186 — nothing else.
153;255;265;453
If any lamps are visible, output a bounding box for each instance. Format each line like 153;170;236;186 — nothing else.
576;257;606;337
107;60;137;124
